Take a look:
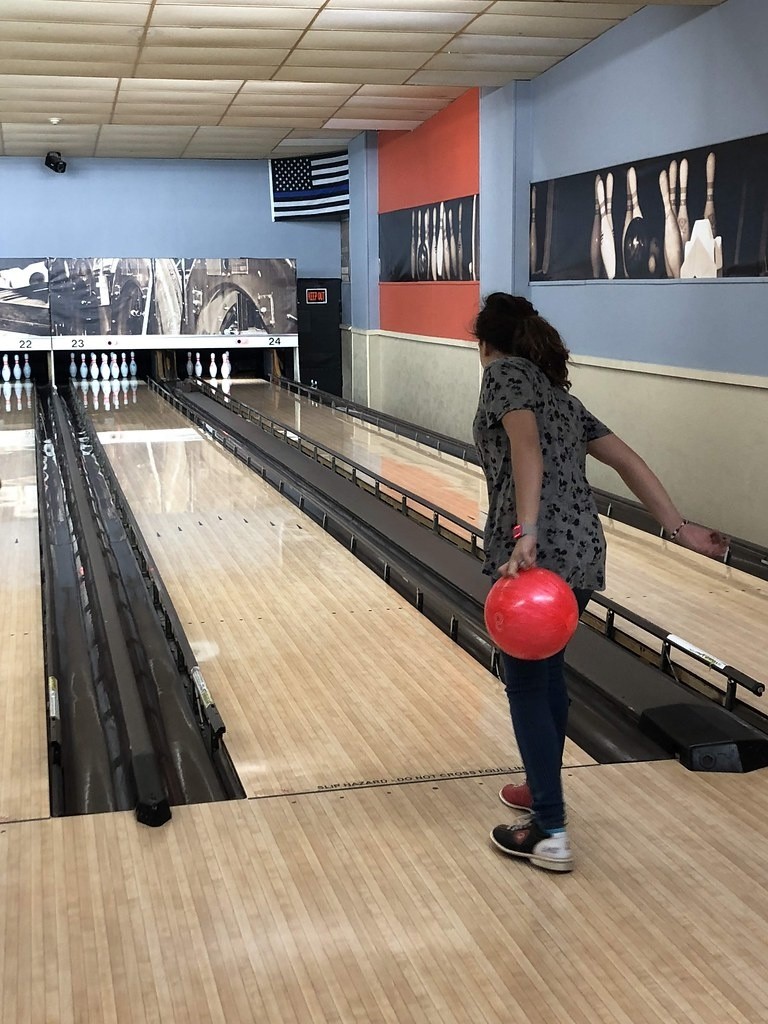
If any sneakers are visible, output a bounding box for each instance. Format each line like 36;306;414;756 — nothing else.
490;814;574;871
498;777;569;825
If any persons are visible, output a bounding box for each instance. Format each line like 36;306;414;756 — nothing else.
472;291;729;871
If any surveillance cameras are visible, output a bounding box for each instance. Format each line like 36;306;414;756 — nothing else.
44;155;67;173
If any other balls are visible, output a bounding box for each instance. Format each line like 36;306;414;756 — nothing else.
484;567;579;660
624;217;651;278
418;242;428;279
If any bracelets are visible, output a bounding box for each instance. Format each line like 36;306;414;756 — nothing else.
670;519;689;543
513;523;538;541
